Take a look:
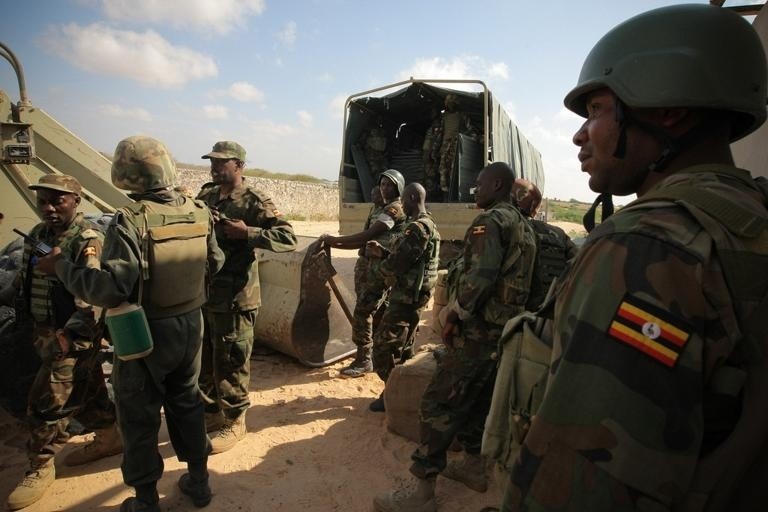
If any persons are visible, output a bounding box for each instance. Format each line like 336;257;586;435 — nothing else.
352;92;483;204
490;0;768;509
6;171;117;511
317;169;440;412
192;141;297;458
30;135;218;511
370;161;575;509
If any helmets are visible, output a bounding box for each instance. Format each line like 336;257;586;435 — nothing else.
380;169;404;196
111;135;176;190
443;93;462;110
508;179;542;218
564;3;768;144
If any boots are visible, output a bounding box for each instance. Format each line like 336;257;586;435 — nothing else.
340;356;373;376
374;475;437;512
438;455;489;494
206;410;247;455
370;386;402;412
120;492;160;512
192;408;225;432
62;425;121;466
6;457;56;510
178;473;211;507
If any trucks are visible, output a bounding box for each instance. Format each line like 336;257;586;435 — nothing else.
332;76;543;271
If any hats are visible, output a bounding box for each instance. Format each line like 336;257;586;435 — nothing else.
202;141;246;162
28;174;82;197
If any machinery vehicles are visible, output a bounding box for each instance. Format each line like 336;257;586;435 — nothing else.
0;35;384;386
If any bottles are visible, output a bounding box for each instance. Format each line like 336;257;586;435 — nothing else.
106;301;155;364
